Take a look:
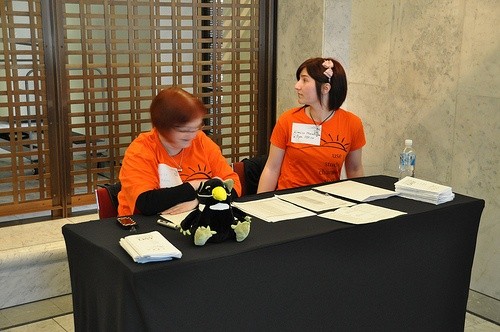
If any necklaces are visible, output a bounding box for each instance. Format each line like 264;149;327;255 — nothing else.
163;146;185;171
307;107;335;136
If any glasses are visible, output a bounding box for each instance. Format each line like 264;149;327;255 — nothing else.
168;123;206;133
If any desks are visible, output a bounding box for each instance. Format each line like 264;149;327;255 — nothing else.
61;174;485;332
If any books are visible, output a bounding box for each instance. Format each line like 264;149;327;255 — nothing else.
117;231;182;264
393;176;456;206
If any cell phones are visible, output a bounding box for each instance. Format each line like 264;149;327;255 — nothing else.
116;216;138;230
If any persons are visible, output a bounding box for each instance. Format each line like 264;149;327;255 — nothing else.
118;86;242;220
257;56;365;198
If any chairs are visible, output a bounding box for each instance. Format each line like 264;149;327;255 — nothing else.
96;182;122;220
230;154;284;194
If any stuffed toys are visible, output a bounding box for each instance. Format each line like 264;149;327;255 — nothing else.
177;176;252;247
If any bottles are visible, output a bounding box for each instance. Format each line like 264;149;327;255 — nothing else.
399;139;416;181
